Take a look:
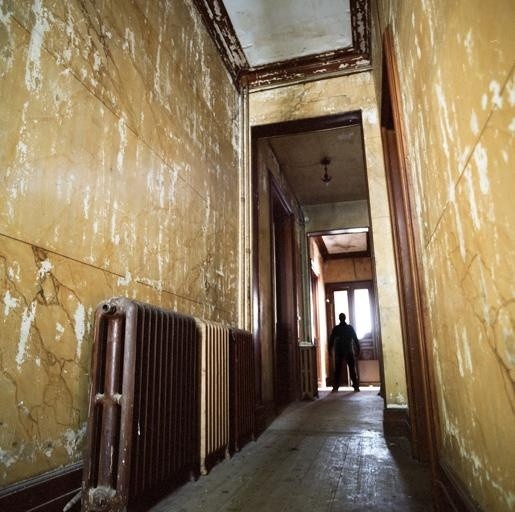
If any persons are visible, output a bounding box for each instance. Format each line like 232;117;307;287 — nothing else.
328;313;361;393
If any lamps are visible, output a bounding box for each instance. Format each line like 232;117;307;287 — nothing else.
320;158;332;186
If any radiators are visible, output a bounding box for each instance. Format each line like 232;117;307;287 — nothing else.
299;341;319;401
